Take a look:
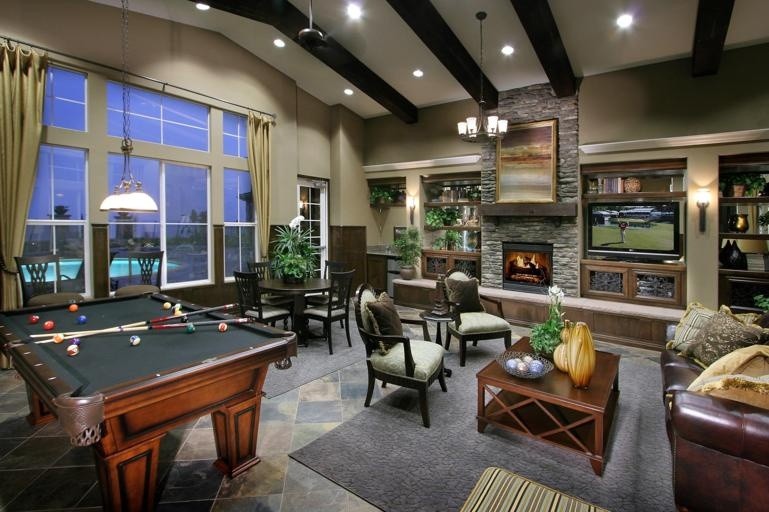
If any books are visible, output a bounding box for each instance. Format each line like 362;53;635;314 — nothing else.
598;178;624;194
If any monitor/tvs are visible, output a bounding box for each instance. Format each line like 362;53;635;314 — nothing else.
587;201;680;264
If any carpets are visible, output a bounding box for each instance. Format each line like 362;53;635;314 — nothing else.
456;466;612;511
283;335;677;511
260;314;369;402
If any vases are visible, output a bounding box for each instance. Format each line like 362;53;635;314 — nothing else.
735;212;749;233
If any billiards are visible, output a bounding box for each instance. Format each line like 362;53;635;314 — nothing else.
29;302;227;357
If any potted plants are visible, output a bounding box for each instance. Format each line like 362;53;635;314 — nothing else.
444;229;463;252
384;228;425;281
731;173;765;197
266;224;323;285
368;190;392;206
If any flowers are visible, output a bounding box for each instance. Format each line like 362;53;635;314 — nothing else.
528;282;573;359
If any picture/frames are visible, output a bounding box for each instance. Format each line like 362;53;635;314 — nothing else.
492;116;561;204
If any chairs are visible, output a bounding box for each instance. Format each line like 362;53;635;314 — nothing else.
111;248;169;297
244;259;295;332
303;267;359;356
13;245;86;312
231;269;292;334
350;280;451;429
304;258;351;330
438;265;515;368
59;247;127;293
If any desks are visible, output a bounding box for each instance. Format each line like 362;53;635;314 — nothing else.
1;288;300;511
419;308;455;348
255;275;342;348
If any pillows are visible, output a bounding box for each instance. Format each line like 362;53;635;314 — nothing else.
364;290;405;355
443;276;487;314
663;300;769;410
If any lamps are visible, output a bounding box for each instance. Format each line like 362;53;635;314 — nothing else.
454;9;509;146
694;187;710;232
409;204;417;225
97;1;162;216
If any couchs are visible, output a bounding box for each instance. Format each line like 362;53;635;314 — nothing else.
658;305;769;511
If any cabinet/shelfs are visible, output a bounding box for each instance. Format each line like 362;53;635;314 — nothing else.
365;252;403;297
580;161;686;310
419;172;482;286
718;155;769;316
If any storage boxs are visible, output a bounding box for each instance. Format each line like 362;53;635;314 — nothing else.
745;253;769;273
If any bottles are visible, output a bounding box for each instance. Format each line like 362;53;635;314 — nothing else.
425;185;479;227
733;214;748;234
726;214;739;234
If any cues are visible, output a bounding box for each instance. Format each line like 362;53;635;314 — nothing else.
29;302;254;344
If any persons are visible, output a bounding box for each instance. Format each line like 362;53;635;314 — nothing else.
619;221;629;240
